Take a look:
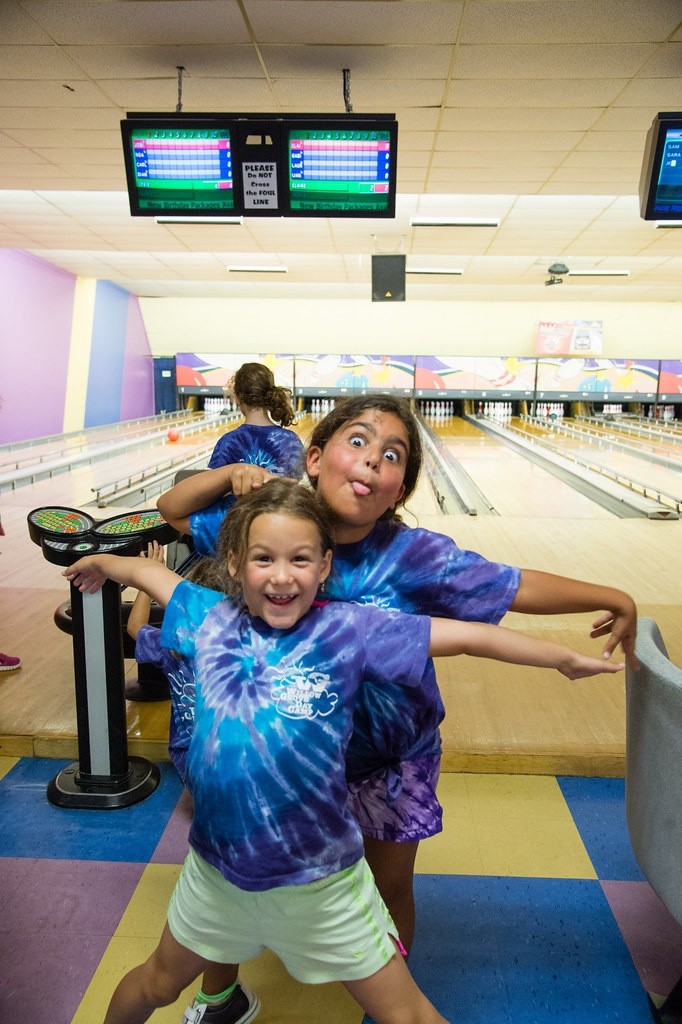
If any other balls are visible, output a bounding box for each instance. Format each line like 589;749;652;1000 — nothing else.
168;430;180;442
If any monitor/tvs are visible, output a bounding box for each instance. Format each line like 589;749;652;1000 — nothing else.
120;119;242;217
638;119;682;222
280;119;399;219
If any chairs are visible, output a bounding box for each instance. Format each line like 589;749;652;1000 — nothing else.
624;619;682;928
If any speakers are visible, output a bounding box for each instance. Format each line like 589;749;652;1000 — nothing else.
371;256;406;302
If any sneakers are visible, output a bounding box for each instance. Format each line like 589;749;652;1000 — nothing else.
180;980;261;1024
0;653;21;671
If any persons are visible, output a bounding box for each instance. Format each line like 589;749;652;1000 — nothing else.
157;394;637;1024
207;363;307;482
126;541;195;792
61;480;625;1024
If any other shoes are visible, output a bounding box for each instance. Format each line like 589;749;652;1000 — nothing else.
125;678;171;701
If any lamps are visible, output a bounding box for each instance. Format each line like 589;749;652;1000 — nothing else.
654;220;682;228
410;216;498;227
405;268;463;275
154;216;245;225
229;266;288;273
